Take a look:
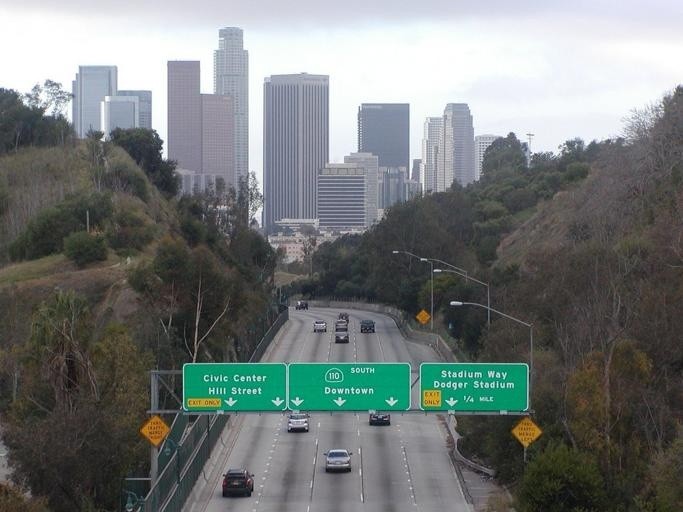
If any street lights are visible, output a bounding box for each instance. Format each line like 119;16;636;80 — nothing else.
124;490;147;512
393;250;433;330
450;300;533;410
420;257;468;285
229;292;288;363
433;268;490;329
165;438;183;485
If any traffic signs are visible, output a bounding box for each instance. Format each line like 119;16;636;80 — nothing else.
183;365;285;410
289;364;410;410
420;364;528;410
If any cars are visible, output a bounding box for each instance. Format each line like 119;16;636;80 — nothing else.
323;448;353;472
286;414;313;433
368;413;391;425
335;332;349;343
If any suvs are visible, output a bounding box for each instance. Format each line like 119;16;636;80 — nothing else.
221;469;255;495
335;319;348;332
338;312;350;322
314;320;327;332
295;301;308;311
360;319;375;333
